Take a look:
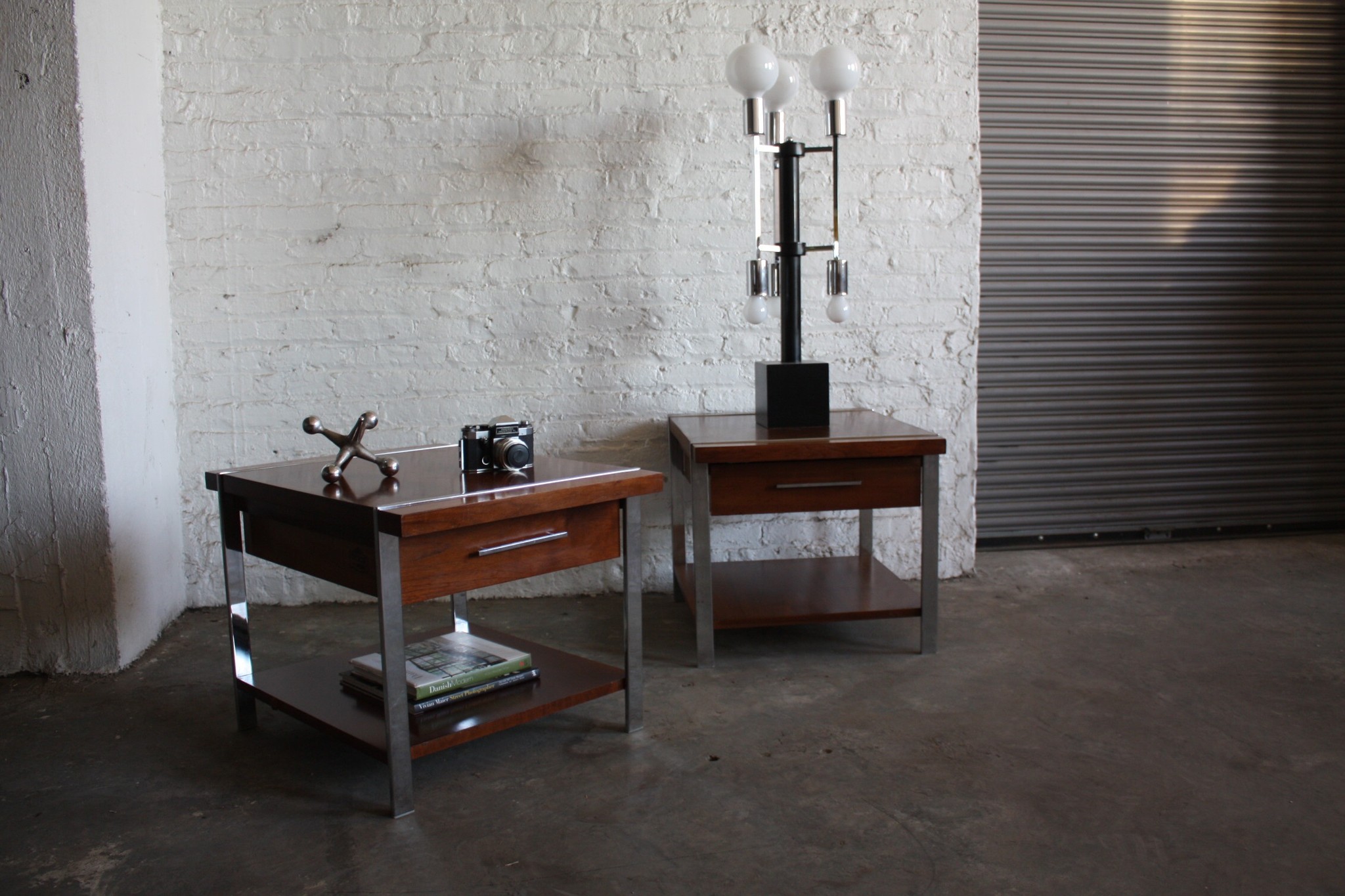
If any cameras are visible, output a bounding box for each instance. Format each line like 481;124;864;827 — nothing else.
459;420;536;474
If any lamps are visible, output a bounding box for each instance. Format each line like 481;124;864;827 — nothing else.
726;42;860;429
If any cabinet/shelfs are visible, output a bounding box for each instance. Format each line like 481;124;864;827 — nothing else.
205;443;662;819
669;408;948;667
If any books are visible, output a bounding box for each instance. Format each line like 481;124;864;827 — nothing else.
338;631;541;714
